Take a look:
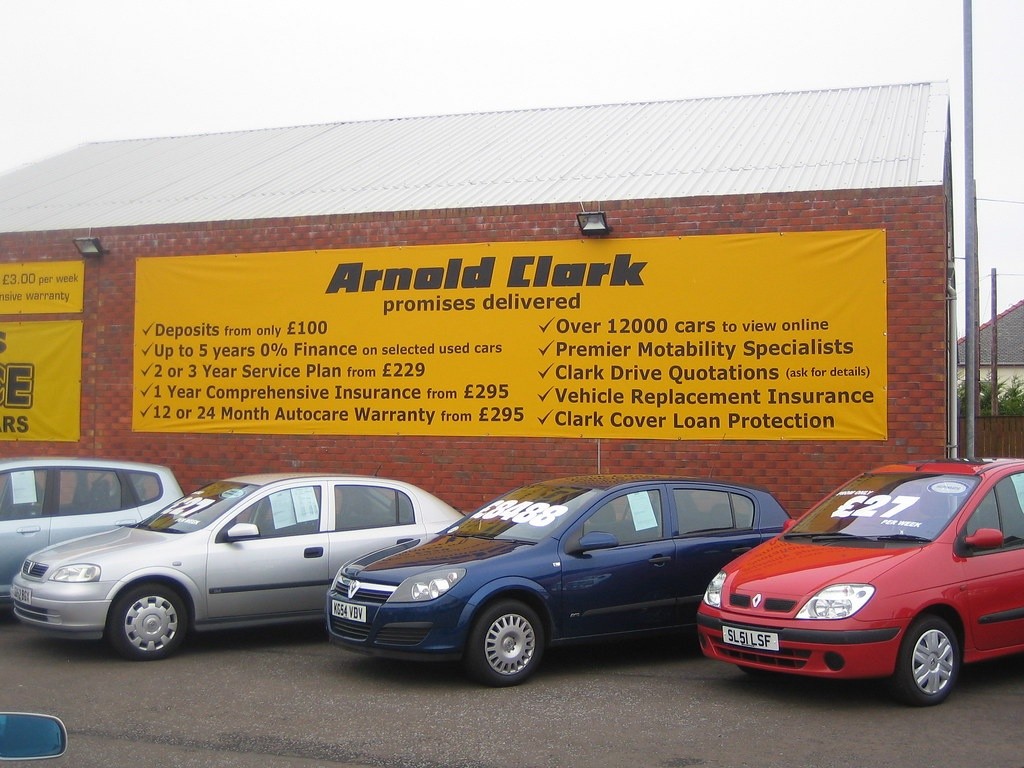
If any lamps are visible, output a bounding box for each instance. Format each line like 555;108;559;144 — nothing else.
576;211;612;236
72;236;110;257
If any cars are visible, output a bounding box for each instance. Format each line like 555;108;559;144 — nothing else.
0;458;188;610
324;473;793;689
10;471;468;663
695;457;1024;707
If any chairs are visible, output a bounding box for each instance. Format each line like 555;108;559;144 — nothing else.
588;501;660;546
915;493;946;534
13;481;43;519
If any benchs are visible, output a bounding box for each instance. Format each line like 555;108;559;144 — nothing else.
676;501;752;533
81;479;121;514
339;497;412;528
